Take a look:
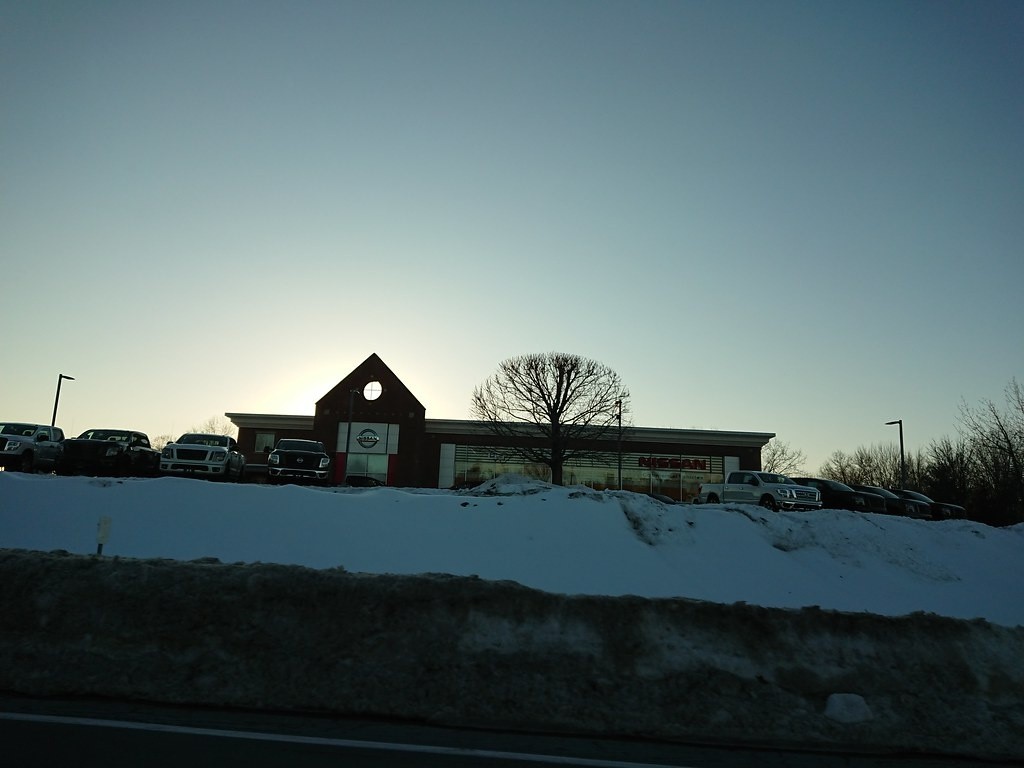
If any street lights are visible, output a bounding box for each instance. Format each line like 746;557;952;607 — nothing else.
52;373;75;427
885;419;907;490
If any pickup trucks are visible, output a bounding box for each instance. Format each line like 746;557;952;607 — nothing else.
691;470;824;513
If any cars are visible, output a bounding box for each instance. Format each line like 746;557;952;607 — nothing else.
158;433;247;482
849;484;933;521
0;422;66;473
783;477;888;515
887;488;968;521
263;438;336;487
55;429;170;478
